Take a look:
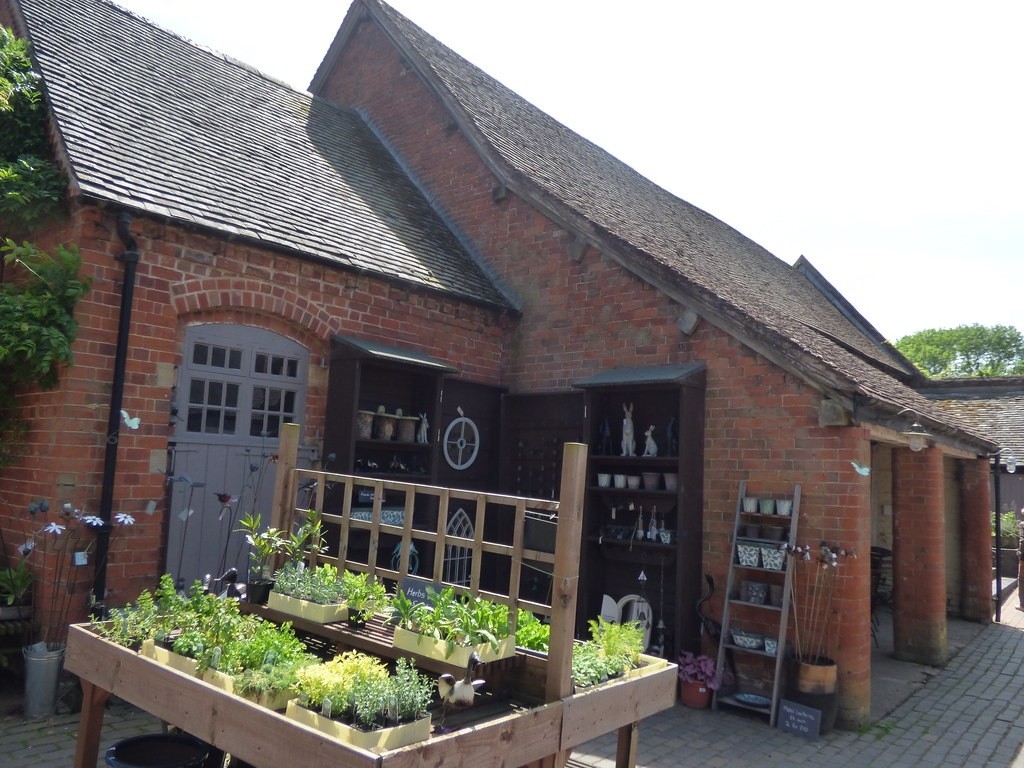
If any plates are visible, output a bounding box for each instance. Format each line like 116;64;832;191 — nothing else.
734;693;771;707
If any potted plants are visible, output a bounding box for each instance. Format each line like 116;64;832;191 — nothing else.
383;584;515;668
571;615;645;693
232;511;291;604
0;556;34;621
268;562;374;629
89;573;438;753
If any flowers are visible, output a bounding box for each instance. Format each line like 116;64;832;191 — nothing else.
19;499;136;651
673;652;728;690
176;430;335;591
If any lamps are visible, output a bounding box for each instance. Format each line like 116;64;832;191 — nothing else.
983;448;1016;473
891;408;933;452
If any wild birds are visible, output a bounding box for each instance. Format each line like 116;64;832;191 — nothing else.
436;651;486;733
214;567;247;602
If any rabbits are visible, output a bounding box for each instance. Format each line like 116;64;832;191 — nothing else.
620;402;636;456
417;412;429;443
643;425;658;456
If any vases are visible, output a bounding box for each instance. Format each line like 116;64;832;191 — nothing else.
614;474;627;488
351;507;406;525
732;631;763;648
769;525;784;541
398;417;419;442
377;414;400;441
793;654;837;694
660;530;676;544
765;638;778;653
740;581;783;606
738;544;761;566
597;474;611;487
628;476;640;489
105;733;215;768
358;411;375;439
761;548;786;570
742;497;792;516
664;473;678;491
641;472;660;491
682;678;711;708
745;524;761;538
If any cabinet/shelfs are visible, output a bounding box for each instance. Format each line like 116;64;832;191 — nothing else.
322;336;461;579
713;480;801;728
573;363;707;665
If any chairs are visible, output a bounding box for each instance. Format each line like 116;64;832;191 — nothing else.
870;546;894;648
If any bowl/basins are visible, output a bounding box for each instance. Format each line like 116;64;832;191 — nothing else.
732;632;764;649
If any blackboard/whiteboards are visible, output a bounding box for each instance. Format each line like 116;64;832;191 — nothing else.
778;700;822;743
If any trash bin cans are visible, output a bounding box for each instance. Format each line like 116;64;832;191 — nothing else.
105;733;210;768
